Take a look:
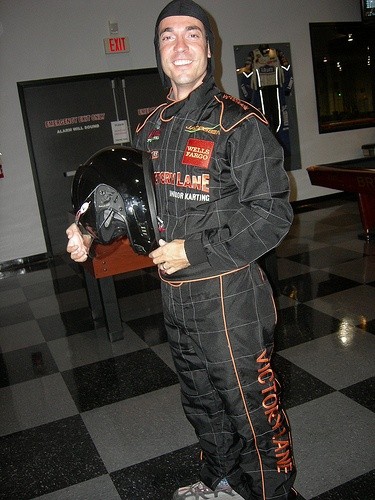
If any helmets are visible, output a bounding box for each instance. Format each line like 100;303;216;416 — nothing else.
71;146;161;255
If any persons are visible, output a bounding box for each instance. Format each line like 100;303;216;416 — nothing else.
62;1;303;500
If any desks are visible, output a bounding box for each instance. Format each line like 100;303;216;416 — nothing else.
66;211;158;342
306;157;375;242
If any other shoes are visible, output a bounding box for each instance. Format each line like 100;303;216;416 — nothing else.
173;478;246;500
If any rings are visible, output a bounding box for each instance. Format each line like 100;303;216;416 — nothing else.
162;263;167;270
